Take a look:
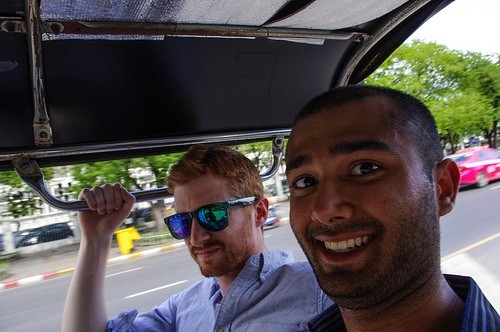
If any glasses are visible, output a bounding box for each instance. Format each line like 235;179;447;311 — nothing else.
162;196;256;241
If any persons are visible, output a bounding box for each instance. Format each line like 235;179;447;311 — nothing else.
284;86;500;332
60;143;345;332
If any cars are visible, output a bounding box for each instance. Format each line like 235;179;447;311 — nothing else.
444;146;500;190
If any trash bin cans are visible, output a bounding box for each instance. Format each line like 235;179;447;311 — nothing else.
114;226;140;255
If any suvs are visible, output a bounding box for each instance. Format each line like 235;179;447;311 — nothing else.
16;222;75;249
